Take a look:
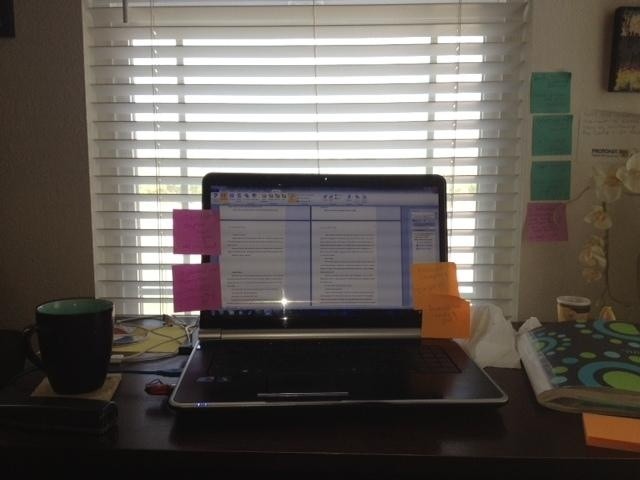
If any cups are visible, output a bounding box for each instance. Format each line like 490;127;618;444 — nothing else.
555;295;592;323
23;298;117;394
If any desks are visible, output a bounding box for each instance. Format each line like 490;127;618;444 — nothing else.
0;315;640;479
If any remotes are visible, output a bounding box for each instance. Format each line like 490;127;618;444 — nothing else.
0;394;120;434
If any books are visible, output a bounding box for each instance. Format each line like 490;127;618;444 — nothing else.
110;314;197;363
515;317;639;417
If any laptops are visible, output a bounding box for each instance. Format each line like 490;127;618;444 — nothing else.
168;170;509;425
